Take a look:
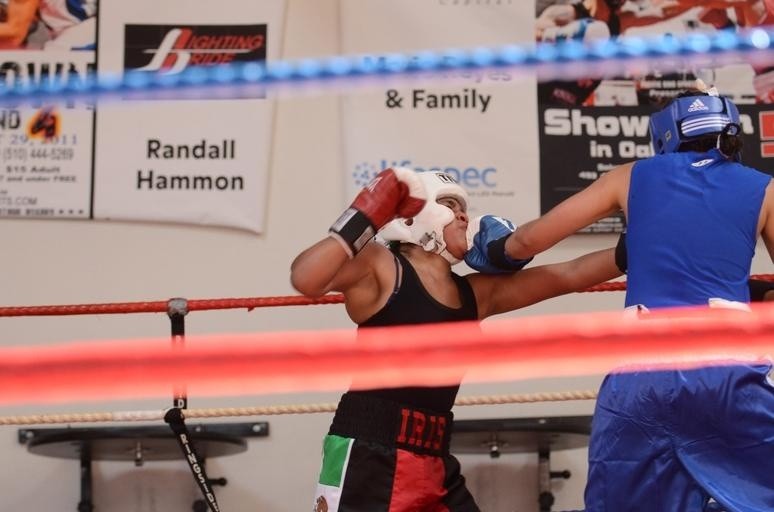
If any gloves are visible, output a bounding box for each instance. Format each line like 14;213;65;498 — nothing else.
465;214;533;274
542;18;610;45
329;166;428;261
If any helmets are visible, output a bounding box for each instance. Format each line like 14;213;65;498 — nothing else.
647;94;741;155
379;170;469;246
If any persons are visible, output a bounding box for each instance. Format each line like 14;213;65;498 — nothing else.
534;0;700;107
455;77;773;511
286;167;626;510
700;1;772;105
